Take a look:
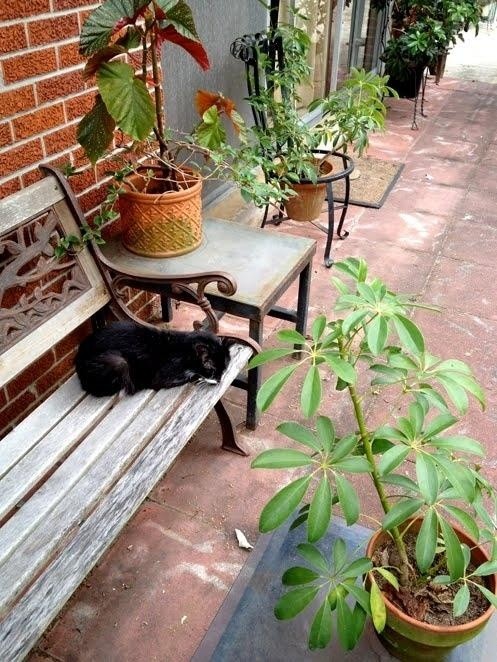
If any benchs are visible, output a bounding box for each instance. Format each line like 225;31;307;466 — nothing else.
0;164;261;662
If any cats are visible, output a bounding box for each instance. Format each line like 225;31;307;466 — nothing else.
74;306;236;395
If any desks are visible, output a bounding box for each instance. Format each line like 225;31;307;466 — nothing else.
99;218;318;431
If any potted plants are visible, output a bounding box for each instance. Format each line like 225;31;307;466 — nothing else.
369;0;490;100
71;1;250;257
248;253;496;662
198;21;400;223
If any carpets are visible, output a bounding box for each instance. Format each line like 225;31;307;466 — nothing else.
185;502;497;662
